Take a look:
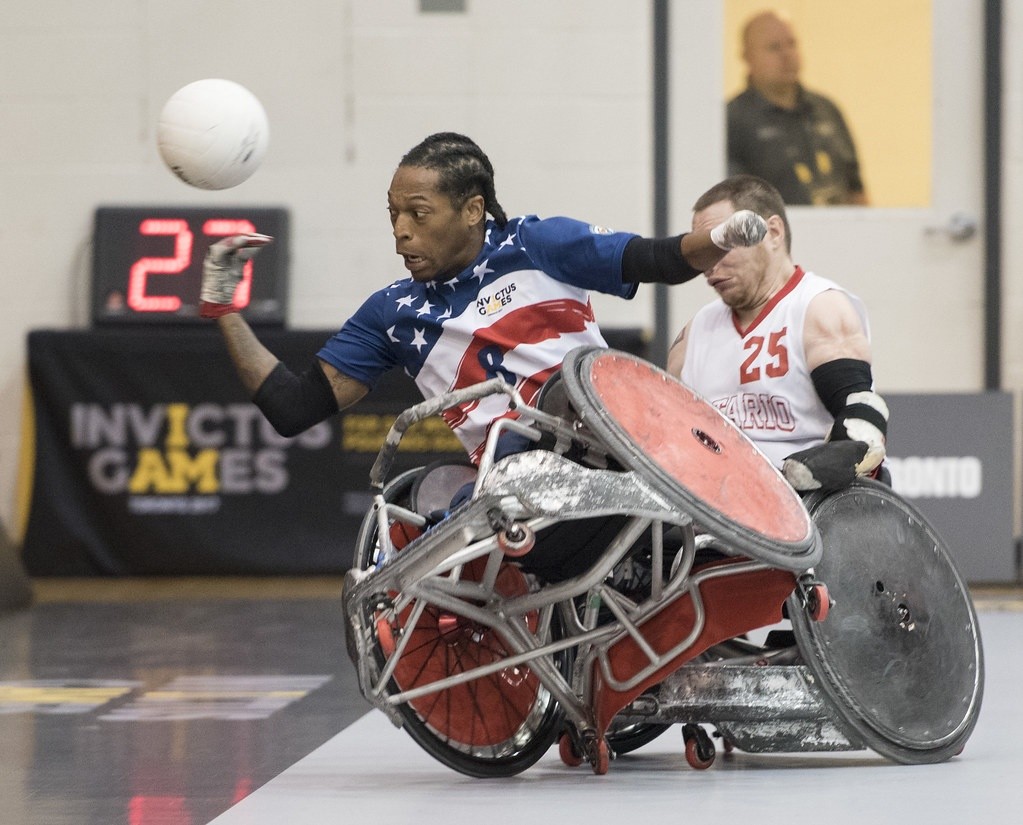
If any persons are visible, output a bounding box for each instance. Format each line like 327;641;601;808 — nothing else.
199;132;767;607
666;174;888;499
728;8;870;206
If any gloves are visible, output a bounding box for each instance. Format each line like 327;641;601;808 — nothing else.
710;209;768;252
198;233;274;318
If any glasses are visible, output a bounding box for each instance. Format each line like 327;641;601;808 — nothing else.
749;39;796;52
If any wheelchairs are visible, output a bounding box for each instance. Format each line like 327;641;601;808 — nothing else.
560;475;984;767
341;340;829;780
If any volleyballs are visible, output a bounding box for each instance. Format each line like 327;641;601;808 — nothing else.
154;77;273;193
